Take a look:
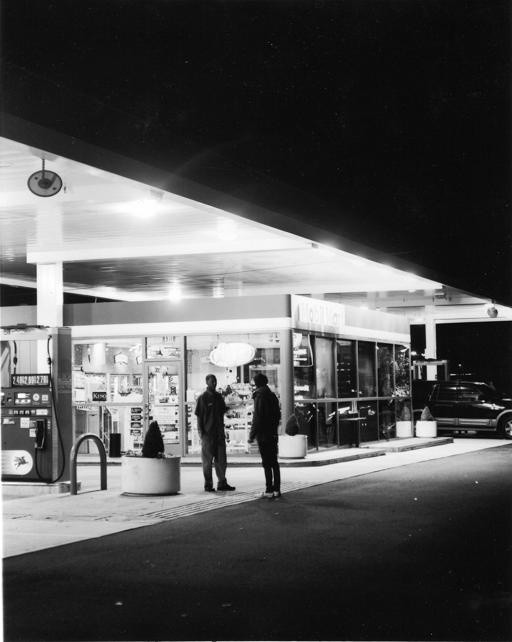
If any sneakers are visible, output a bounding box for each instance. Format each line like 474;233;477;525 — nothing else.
205;484;235;492
257;490;281;498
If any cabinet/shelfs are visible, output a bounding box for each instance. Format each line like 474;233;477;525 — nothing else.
222;398;254;430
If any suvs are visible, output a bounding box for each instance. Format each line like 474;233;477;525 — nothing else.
412;379;512;439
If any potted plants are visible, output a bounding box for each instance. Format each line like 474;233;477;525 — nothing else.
121;419;183;495
415;405;438;438
276;413;309;459
395;406;413;439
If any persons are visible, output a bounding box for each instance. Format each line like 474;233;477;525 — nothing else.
194;373;236;492
244;373;282;498
